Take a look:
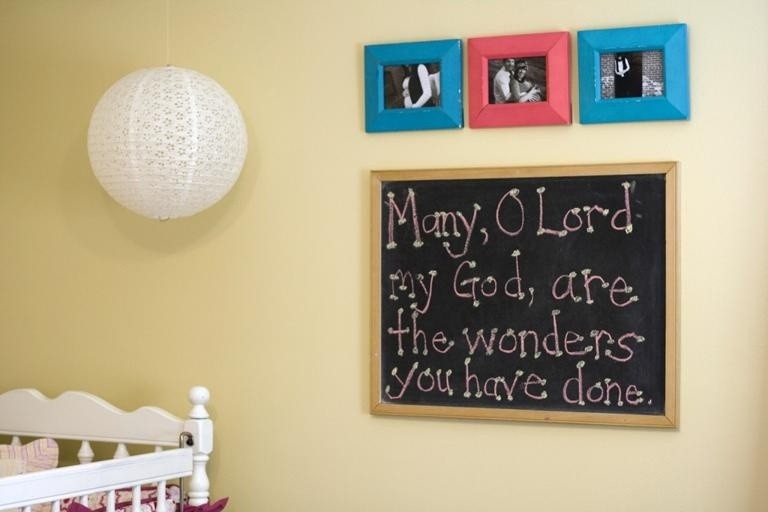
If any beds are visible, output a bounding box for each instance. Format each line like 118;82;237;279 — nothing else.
0;385;229;512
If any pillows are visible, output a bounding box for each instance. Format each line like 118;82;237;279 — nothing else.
1;438;60;477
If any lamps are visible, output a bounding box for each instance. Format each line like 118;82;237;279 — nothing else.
87;0;247;218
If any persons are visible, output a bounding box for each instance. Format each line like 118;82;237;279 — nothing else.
615;54;633;96
401;63;432;108
493;58;537;104
511;59;542;102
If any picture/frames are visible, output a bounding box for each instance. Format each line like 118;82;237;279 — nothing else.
365;39;463;133
467;30;571;129
578;22;690;125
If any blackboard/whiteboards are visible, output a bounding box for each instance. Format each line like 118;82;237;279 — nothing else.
368;161;678;430
